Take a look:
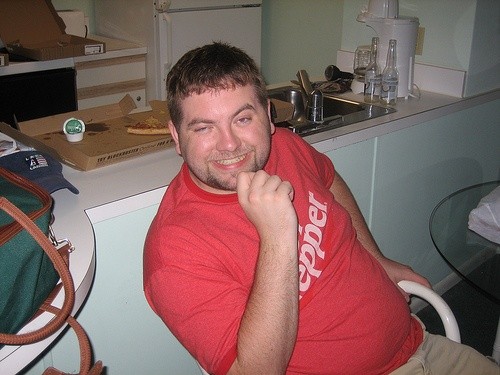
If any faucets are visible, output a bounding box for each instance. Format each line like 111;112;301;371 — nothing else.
296;69;324;122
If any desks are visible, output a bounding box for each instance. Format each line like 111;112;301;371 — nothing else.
428;179;500;301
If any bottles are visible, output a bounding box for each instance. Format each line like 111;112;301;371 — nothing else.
381;39;399;106
365;31;382;102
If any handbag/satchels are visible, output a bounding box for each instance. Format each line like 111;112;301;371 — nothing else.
0;166;102;375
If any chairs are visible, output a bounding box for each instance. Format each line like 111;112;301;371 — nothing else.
197;279;461;375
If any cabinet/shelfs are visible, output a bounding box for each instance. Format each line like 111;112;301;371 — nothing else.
18;89;500;375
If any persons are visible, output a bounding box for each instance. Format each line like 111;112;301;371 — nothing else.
143;42;500;375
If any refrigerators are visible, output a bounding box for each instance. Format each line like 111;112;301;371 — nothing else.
95;0;262;106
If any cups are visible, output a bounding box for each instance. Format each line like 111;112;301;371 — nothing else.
354;45;371;81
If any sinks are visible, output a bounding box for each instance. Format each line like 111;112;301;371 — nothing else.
264;84;399;137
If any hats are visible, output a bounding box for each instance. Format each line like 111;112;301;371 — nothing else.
0;151;79;194
